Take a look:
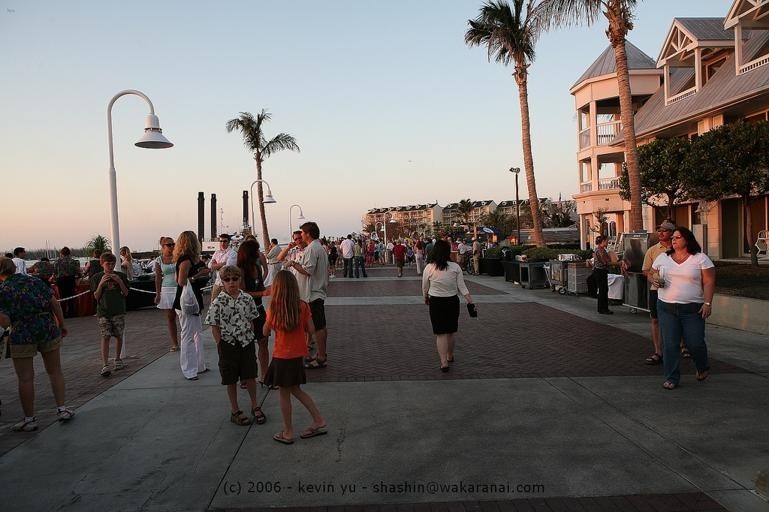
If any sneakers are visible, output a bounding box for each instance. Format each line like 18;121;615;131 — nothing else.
57;409;75;419
600;310;614;315
113;359;124;369
9;416;38;432
101;366;111;375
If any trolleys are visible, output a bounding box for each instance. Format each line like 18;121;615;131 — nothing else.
619;232;653;318
544;259;568;294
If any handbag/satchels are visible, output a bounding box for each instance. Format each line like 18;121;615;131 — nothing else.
0;330;10;359
467;302;477;318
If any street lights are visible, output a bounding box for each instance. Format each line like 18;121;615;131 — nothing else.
289;203;305;242
106;85;174;279
370;211;397;266
508;167;521;247
250;177;276;242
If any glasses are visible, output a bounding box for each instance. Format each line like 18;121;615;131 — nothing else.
164;244;175;247
223;277;239;281
669;236;683;239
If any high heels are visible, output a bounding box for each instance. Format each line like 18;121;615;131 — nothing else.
240;383;247;389
259;381;279;390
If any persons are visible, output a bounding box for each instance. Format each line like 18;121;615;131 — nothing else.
642;220;694;365
647;226;716;391
592;235;622;314
0;222;500;445
586;249;600;297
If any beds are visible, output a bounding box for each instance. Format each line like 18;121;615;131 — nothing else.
45;277;96;319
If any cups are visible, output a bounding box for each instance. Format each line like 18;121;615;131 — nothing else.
656;268;665;288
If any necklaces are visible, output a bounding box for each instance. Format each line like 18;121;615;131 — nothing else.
672;254;689;263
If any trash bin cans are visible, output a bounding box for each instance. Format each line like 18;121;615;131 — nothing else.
607;274;624;299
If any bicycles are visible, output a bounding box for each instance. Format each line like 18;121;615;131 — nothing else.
463;251;482;277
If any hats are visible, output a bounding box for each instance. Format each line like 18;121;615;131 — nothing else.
656;220;675;230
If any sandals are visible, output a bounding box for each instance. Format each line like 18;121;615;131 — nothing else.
681;345;692;359
251;407;266;424
644;353;664;364
304;352;327;368
231;410;250;425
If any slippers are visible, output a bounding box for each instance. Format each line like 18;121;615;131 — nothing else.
662;381;676;389
273;431;293;443
440;366;449;372
170;345;178;351
300;427;328;438
446;355;454;362
695;369;710;384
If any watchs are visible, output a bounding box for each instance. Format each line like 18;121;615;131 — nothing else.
704;302;712;306
